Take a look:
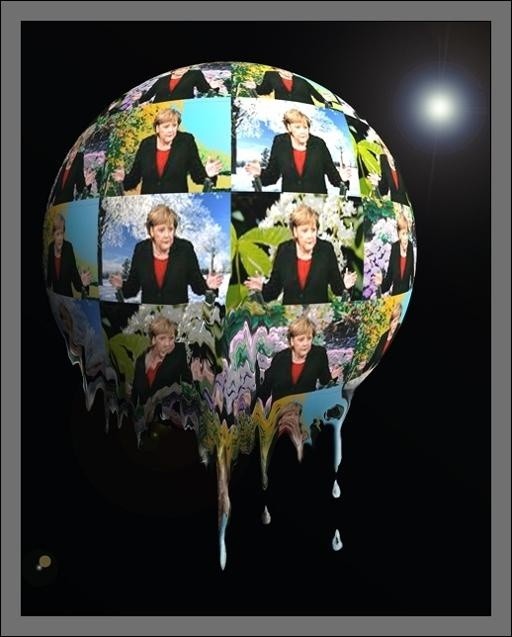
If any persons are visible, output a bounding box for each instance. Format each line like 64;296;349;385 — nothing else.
274;402;306;463
130;316;193;408
58;304;75;338
371;214;416;299
131;65;223;111
114;105;213;192
245;65;329;109
44;213;91;298
358;303;404;377
47;135;92;205
257;311;332;399
308;419;320;443
111;204;224;302
318;418;324;430
250;202;360;304
248;108;346;194
